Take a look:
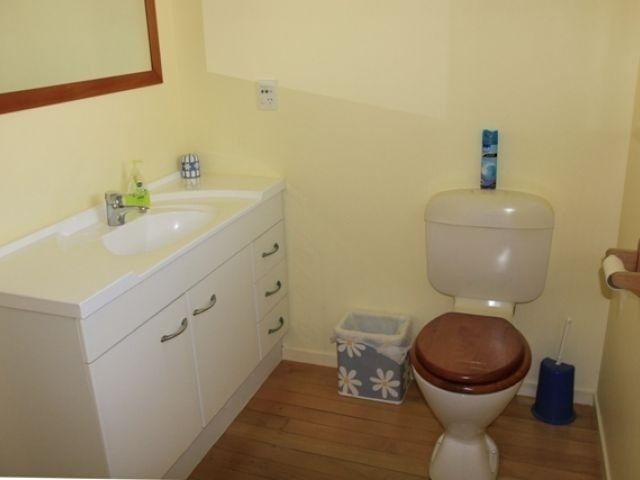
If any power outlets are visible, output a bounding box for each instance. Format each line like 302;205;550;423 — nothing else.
258;86;279;112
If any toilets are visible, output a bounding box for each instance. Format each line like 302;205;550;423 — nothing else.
408;188;555;479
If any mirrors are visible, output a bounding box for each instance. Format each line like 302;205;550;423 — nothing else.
0;0;163;115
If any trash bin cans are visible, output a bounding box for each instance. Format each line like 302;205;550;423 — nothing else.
333;309;415;405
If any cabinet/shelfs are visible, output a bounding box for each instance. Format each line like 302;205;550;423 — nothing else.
252;219;290;360
81;250;261;480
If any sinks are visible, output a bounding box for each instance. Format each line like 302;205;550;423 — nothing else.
100;204;219;253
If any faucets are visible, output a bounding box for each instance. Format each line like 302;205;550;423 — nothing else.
105;192;149;228
